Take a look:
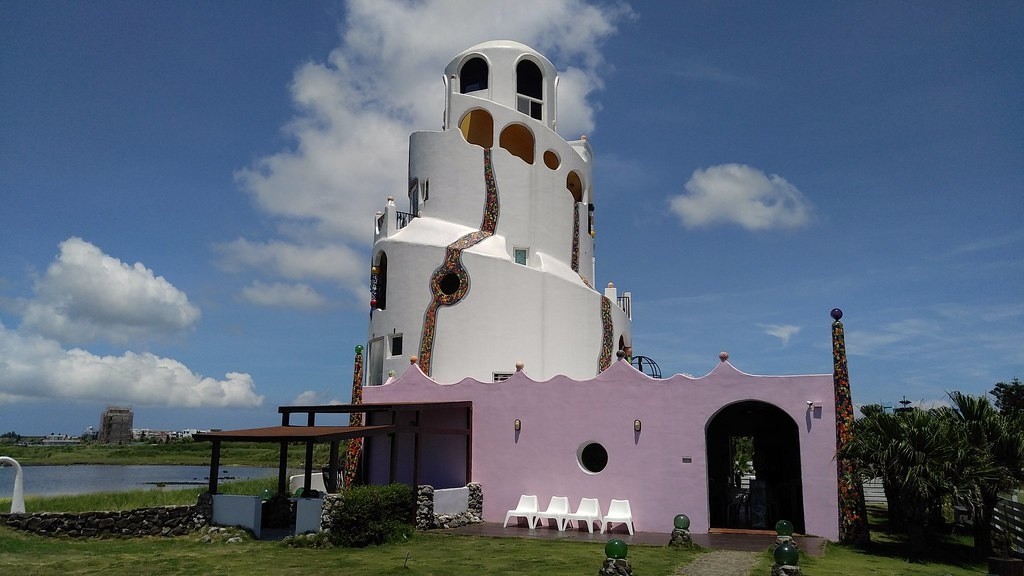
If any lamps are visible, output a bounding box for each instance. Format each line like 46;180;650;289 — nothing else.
515;419;520;431
634;419;641;431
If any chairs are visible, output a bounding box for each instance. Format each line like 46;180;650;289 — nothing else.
534;496;572;530
503;495;539;530
600;499;635;535
562;498;602;533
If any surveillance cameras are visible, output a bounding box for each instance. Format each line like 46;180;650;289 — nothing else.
806;401;813;406
